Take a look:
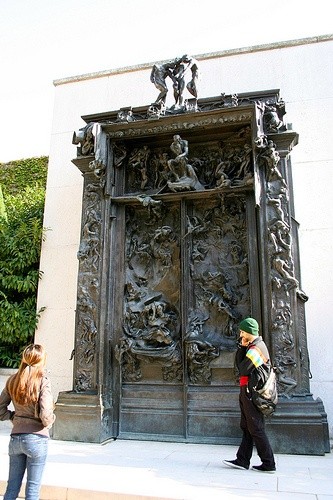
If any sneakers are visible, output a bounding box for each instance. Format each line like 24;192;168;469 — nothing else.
222;458;248;471
251;463;276;474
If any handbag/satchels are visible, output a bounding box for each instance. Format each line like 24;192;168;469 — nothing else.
248;363;278;417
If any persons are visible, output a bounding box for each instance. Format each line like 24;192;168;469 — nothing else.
222;318;277;472
113;54;255;190
0;342;56;500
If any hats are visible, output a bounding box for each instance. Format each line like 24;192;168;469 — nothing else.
239;318;261;337
